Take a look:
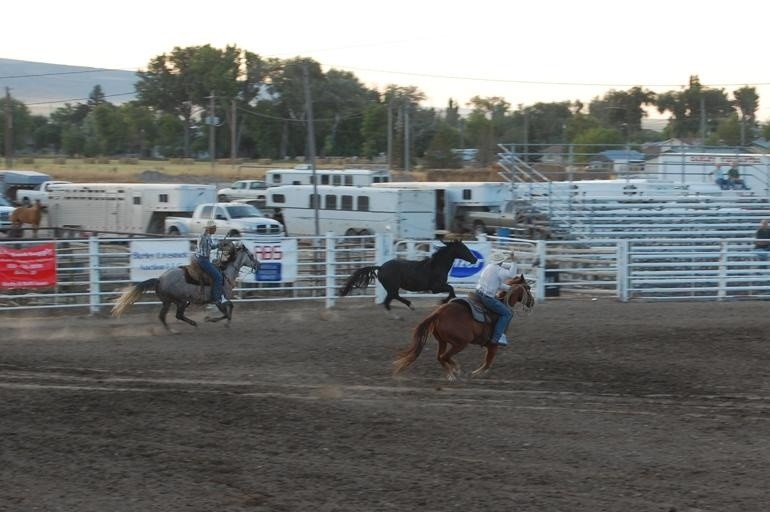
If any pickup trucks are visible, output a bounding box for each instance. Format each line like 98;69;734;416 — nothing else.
463;200;573;241
15;181;72;210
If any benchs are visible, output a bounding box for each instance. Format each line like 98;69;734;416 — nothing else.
514;179;770;239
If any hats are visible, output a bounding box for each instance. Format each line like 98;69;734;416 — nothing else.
488;251;511;265
200;220;221;228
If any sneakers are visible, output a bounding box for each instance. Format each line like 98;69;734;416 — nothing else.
215;299;231;306
491;338;507;346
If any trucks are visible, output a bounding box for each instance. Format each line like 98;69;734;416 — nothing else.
46;181;284;246
228;185;437;247
265;168;391;188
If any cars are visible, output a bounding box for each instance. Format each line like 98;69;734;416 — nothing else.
217;178;279;203
0;194;21;232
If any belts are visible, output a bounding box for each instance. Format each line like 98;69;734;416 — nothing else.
476;288;494;299
195;254;210;259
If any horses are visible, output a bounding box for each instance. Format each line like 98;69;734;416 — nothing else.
10;198;43;238
109;242;259;333
338;237;479;318
390;274;537;382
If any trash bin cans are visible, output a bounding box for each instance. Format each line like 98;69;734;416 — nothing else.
545;263;559;296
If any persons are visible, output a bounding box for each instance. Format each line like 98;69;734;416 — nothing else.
196;218;228;311
474;248;519;345
